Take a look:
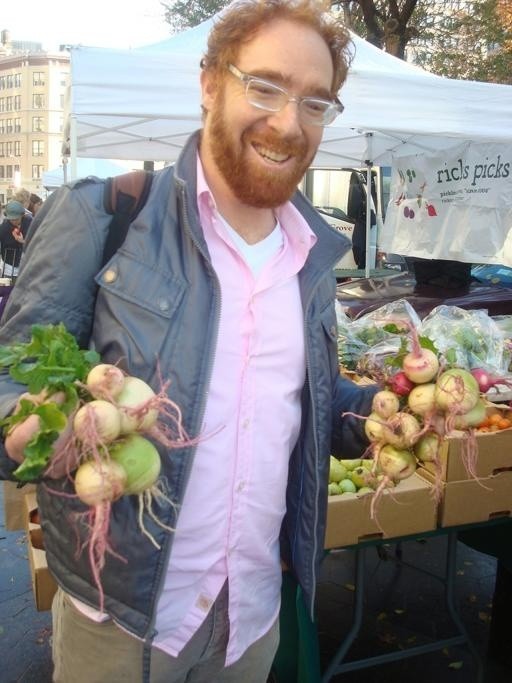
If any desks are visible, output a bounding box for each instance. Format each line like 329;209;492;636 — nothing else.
324;522;512;683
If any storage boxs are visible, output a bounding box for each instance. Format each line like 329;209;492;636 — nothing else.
15;495;67;607
429;430;512;482
442;480;512;533
323;479;442;557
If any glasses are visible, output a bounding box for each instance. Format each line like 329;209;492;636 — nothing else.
229;64;345;126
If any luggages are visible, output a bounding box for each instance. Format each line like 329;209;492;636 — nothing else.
0;248;19;318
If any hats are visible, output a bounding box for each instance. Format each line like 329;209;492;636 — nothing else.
5;201;25;220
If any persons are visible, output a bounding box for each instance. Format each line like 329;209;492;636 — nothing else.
345;170;377;274
1;0;441;681
1;185;44;313
405;250;472;299
